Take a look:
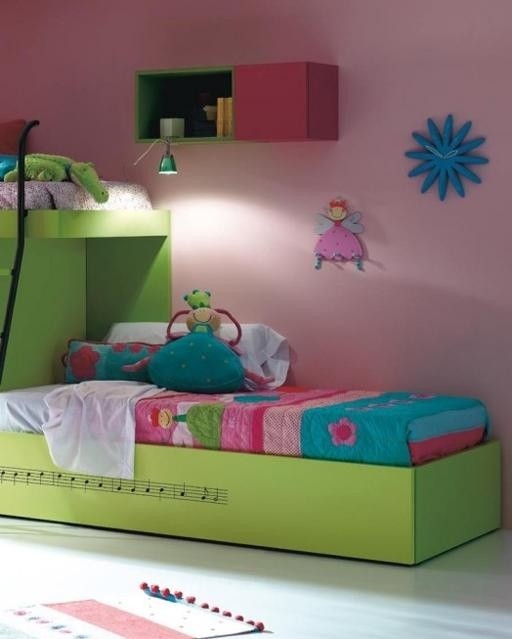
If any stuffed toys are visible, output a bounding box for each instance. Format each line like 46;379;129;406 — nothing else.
4;153;108;203
121;289;275;394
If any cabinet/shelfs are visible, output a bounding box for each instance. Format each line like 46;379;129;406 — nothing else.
135;61;340;144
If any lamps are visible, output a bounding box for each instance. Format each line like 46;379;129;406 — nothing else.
133;137;178;175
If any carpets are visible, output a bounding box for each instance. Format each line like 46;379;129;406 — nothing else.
1;582;275;639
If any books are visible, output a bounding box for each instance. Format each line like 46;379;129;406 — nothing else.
223;96;234;137
216;98;224;136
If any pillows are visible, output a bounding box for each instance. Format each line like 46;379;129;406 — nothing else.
60;320;291;390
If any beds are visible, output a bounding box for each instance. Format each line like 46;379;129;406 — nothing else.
1;383;503;570
